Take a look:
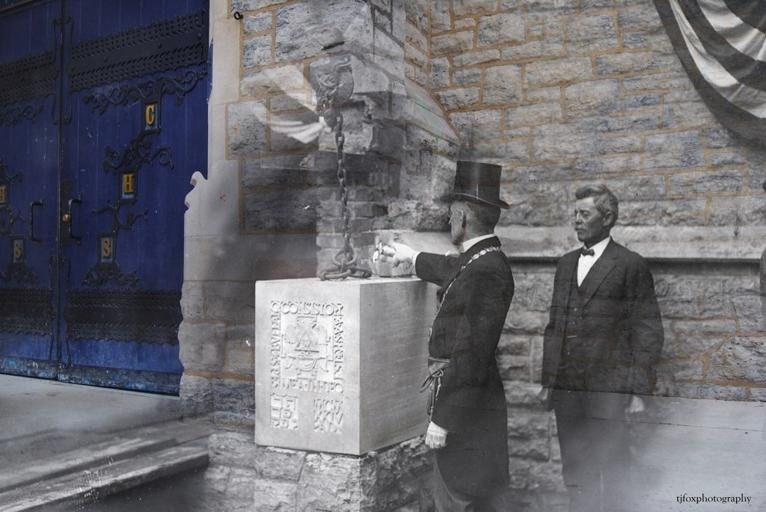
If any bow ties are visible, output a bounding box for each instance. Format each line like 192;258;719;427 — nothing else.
581;247;595;256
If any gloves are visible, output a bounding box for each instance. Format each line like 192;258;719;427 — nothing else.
425;421;448;449
380;241;421;268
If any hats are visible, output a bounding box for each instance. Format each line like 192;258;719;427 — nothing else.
440;161;511;210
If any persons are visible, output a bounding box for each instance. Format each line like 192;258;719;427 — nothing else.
538;182;663;512
378;155;517;512
